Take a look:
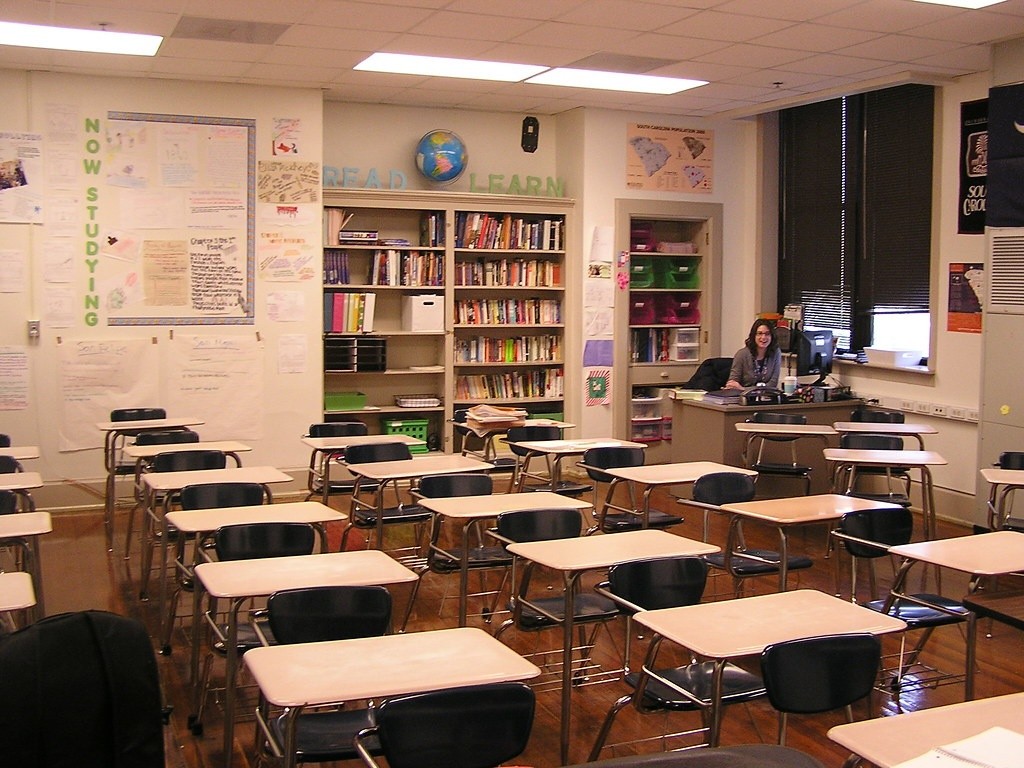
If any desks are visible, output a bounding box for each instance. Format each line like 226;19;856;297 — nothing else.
735;422;838;493
0;512;53;619
462;418;576;482
505;530;721;765
0;447;39;473
518;438;649;513
417;492;595;628
345;454;496;552
302;435;427;530
823;448;948;596
828;691;1023;768
121;441;252;560
600;462;759;550
964;589;1024;630
881;531;1023;702
833;422;939;570
165;501;349;729
980;469;1024;638
0;471;44;513
721;494;905;599
195;550;419;767
633;590;907;749
243;628;542;768
97;419;205;552
0;572;37;632
142;465;294;644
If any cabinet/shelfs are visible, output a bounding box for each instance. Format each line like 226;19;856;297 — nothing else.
670;397;861;502
973;226;1024;537
614;197;723;465
322;188;575;489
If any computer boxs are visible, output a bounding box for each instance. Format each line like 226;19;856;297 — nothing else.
795;386;850;403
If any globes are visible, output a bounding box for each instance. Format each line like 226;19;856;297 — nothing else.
414;128;469;190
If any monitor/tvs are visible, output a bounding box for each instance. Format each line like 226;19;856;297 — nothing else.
798;330;833;386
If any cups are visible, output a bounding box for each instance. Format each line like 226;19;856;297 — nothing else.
784;376;797;395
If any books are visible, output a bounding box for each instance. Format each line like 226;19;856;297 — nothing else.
893;723;1023;768
320;207;669;436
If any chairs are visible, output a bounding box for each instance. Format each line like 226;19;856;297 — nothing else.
681;358;733;391
0;409;1024;768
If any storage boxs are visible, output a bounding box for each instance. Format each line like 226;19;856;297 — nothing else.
630;254;699;289
401;295;444;334
863;347;925;367
326;337;387;373
394;394;441;407
669;328;700;362
325;391;368;410
651;387;673;417
529;412;564;439
630;292;702;324
381;418;429;455
632;223;657;251
814;387;851;402
631;404;673;442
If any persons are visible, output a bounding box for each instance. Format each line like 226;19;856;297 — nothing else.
727;318;782;394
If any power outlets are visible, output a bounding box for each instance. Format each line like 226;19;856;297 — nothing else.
916;403;931;412
868;398;884;406
901;400;914;412
968;412;980;421
951;408;965;418
933;407;948;415
